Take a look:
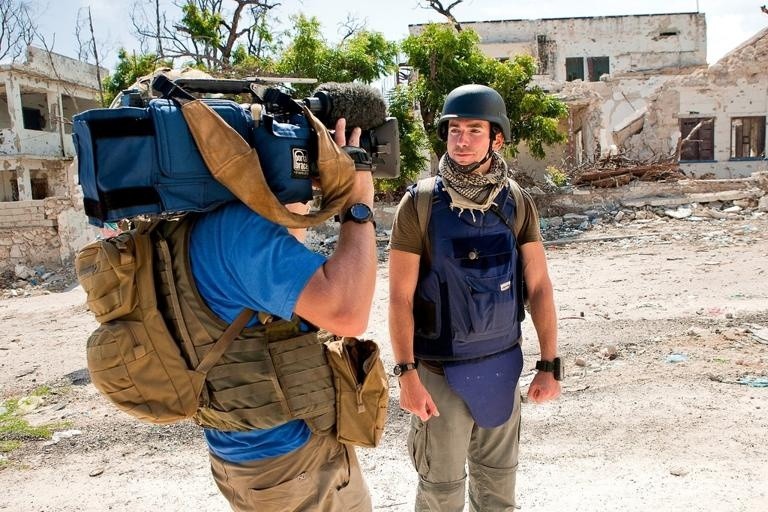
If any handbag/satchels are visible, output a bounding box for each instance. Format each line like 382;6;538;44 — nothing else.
325;336;389;448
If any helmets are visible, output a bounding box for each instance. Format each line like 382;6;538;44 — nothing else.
438;84;512;142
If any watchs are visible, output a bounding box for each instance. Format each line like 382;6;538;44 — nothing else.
394;362;419;376
535;356;564;380
333;202;376;229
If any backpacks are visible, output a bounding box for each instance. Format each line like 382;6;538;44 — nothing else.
75;221;255;423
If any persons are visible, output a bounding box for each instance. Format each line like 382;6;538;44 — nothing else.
73;68;385;512
388;84;562;512
